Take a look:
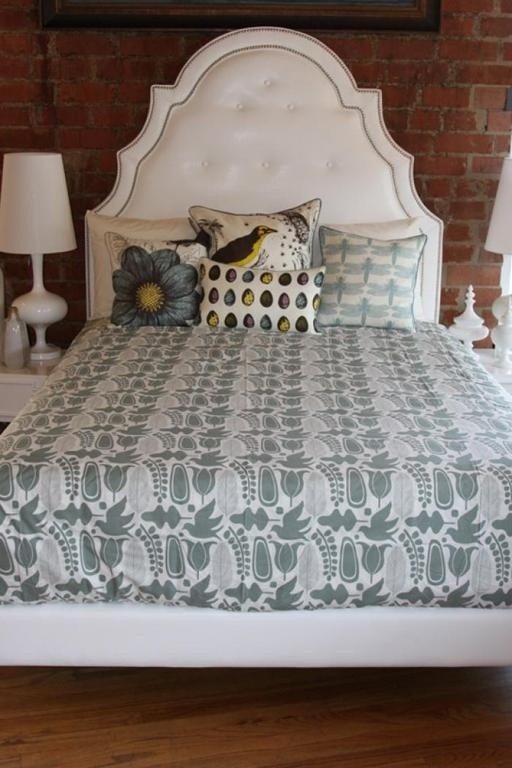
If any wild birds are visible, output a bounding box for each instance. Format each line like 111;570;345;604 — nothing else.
210;225;278;269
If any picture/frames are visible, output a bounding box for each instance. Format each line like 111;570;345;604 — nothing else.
34;0;445;33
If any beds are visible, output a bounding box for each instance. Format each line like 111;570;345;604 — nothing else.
0;25;512;669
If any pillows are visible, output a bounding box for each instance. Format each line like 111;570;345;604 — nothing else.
317;224;428;332
196;254;327;336
85;207;200;322
190;197;325;268
330;219;430;324
102;229;209;327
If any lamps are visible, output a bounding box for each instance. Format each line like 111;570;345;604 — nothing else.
480;154;512;369
0;151;79;355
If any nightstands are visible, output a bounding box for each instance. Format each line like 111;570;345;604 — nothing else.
0;348;67;427
470;345;511;395
